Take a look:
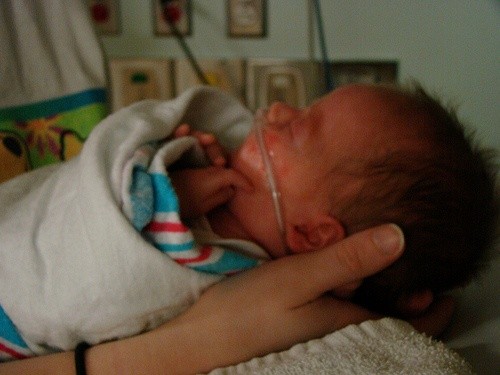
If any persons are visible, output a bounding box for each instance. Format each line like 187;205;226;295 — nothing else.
0;73;500;363
0;224;458;375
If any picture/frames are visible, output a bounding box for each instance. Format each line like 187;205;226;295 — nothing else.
152;0;192;36
85;0;121;35
227;0;267;37
107;56;399;112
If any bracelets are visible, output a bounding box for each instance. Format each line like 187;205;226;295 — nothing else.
74;341;89;374
